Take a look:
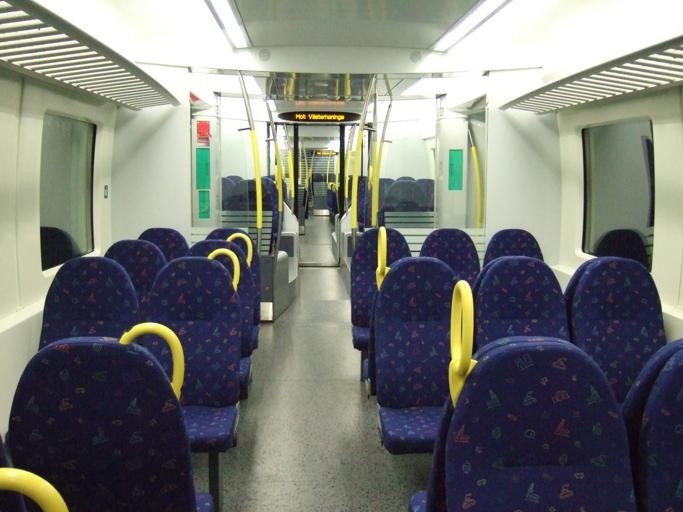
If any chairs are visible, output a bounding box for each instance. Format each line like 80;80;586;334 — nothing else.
330;175;434;265
221;175;301;321
